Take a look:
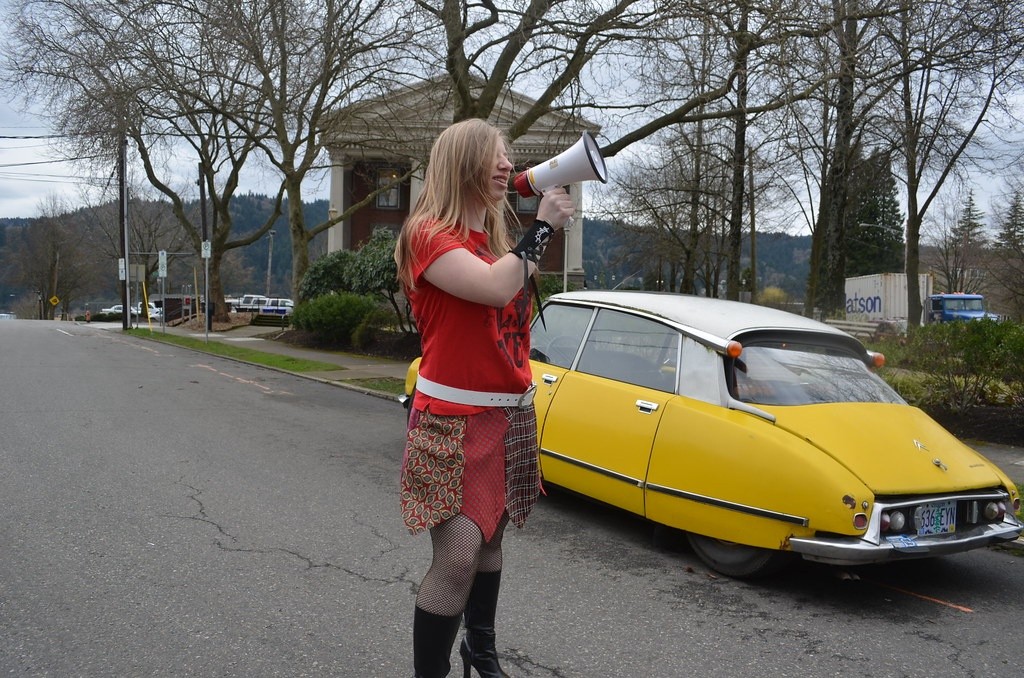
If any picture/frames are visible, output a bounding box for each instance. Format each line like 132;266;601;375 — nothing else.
374;166;400;210
514;168;540;214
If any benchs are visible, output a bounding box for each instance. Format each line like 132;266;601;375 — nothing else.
535;347;661;390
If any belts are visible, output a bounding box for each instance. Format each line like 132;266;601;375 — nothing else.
415;371;538;409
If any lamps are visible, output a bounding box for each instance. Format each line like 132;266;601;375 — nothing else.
328;204;338;228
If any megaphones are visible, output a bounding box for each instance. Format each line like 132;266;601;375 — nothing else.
513;130;608;198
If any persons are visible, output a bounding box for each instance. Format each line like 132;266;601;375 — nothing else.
392;117;575;678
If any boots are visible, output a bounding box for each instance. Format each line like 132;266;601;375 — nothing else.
413;605;462;678
459;571;510;677
